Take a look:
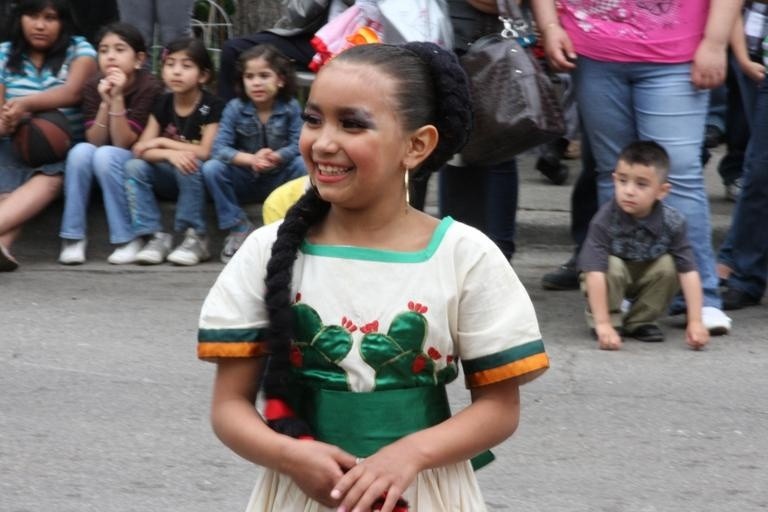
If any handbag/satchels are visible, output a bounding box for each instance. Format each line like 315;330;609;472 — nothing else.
458;33;566;168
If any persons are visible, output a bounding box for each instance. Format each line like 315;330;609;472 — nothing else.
196;43;549;512
436;0;768;349
0;0;336;271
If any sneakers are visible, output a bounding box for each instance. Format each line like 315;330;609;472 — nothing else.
167;227;211;266
107;238;145;265
137;232;172;263
543;259;578;290
59;241;86;264
219;225;256;264
701;306;733;334
634;324;663;340
